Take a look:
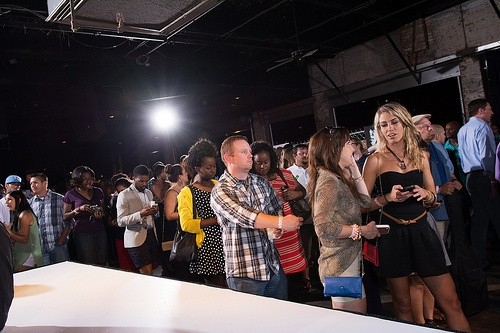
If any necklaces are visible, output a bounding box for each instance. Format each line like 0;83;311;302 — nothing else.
386;139;408;169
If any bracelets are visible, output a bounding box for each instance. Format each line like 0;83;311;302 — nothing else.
425;192;437;206
384;195;389;203
349;224;359;241
357;226;362;240
422;190;430;202
424;192;434;205
352;176;363;182
374;196;388;207
278;215;283;229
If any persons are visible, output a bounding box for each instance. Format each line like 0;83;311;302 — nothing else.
0;98;500;333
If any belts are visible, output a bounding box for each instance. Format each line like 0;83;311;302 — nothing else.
380;209;427;225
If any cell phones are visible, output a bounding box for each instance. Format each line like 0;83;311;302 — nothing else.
281;186;289;193
375;224;390;234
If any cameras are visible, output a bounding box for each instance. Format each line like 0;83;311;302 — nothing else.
90;206;96;211
401;186;414;193
151;204;158;211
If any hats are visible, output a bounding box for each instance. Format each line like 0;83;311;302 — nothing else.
411;114;431;124
5;175;22;184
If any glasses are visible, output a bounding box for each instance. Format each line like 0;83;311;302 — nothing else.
325;125;337;142
80;177;96;182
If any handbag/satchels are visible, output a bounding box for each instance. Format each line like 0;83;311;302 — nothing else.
168;185;198;263
276;168;312;222
362;242;379;267
161;241;173;252
456;268;490;318
323;275;363;298
